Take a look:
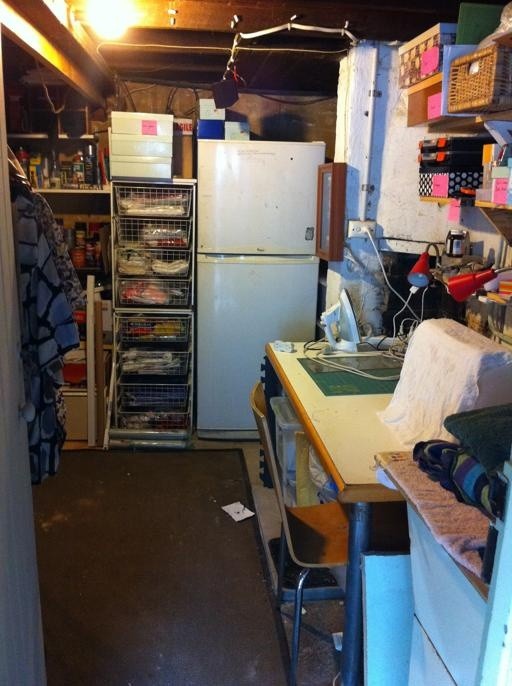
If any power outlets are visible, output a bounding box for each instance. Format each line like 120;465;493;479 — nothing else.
348;220;375;237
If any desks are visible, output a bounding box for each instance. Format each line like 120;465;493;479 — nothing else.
265;338;405;686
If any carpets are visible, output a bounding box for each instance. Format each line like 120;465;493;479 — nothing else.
32;443;294;686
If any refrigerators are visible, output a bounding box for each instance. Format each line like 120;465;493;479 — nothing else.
194;136;328;443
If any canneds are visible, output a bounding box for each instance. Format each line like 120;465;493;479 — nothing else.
70;248;86;267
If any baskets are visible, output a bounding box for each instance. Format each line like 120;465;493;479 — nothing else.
447;43;511;113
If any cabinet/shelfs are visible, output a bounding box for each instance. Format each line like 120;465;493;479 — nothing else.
101;179;200;452
22;175;112;452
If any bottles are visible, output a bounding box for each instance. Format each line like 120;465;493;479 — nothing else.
71;149;84;183
74;221;87;246
445;229;466;258
84;144;97;183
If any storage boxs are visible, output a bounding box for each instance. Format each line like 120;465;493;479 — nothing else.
397;22;456;128
115;414;192;435
115;314;191;346
118;380;189;412
116;217;193;249
116;275;191;308
116;349;191;379
115;247;193;281
114;185;196;219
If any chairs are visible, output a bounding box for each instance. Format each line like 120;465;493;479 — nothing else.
248;380;349;686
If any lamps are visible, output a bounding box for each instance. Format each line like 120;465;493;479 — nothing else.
406;242;512;304
211;21;358;110
